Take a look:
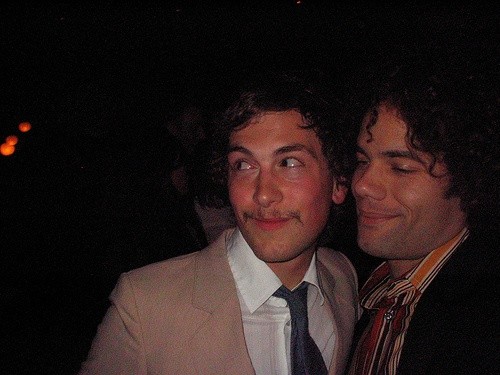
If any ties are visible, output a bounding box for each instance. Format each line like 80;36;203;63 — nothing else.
273;281;332;375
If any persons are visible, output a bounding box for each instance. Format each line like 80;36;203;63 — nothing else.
334;43;500;375
80;69;367;375
139;89;211;271
